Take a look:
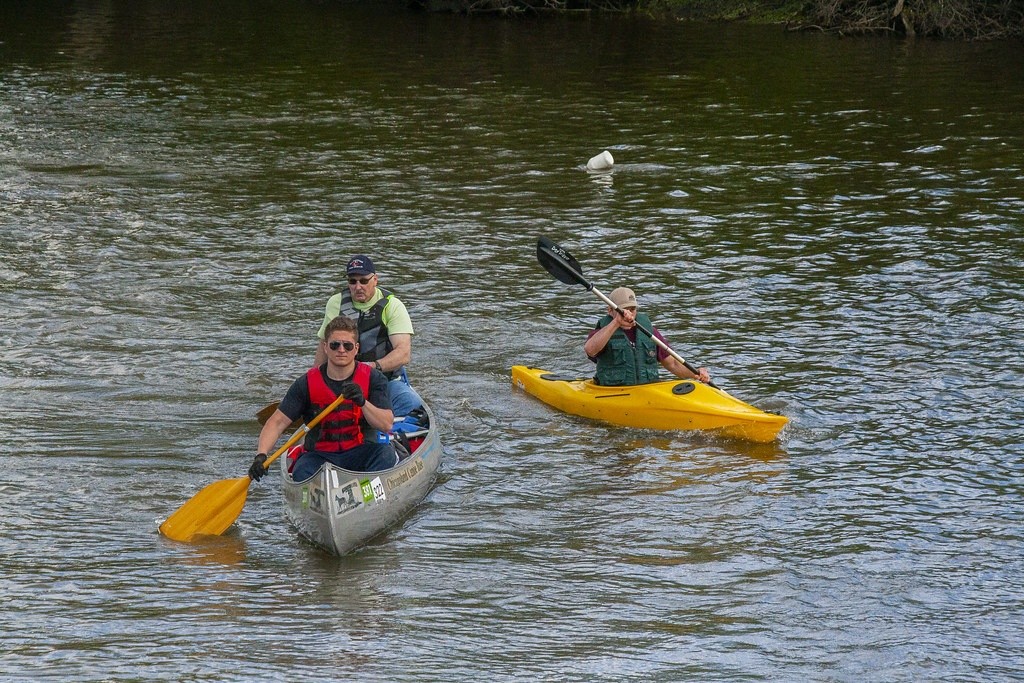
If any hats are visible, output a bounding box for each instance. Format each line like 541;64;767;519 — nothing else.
347;255;374;276
609;287;638;308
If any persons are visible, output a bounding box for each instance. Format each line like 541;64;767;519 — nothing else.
314;254;423;416
248;316;396;483
585;286;710;387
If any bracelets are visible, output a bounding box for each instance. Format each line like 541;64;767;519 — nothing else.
374;361;382;371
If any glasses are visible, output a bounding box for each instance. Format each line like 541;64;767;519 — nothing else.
329;342;354;352
349;275;374;284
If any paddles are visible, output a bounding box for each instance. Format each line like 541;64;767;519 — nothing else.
539;372;593;382
256;401;304;428
535;234;732;397
157;388;348;543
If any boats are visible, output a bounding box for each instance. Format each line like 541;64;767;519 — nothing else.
279;380;443;559
511;363;790;447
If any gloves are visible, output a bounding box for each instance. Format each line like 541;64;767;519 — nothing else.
249;453;269;481
341;381;365;407
